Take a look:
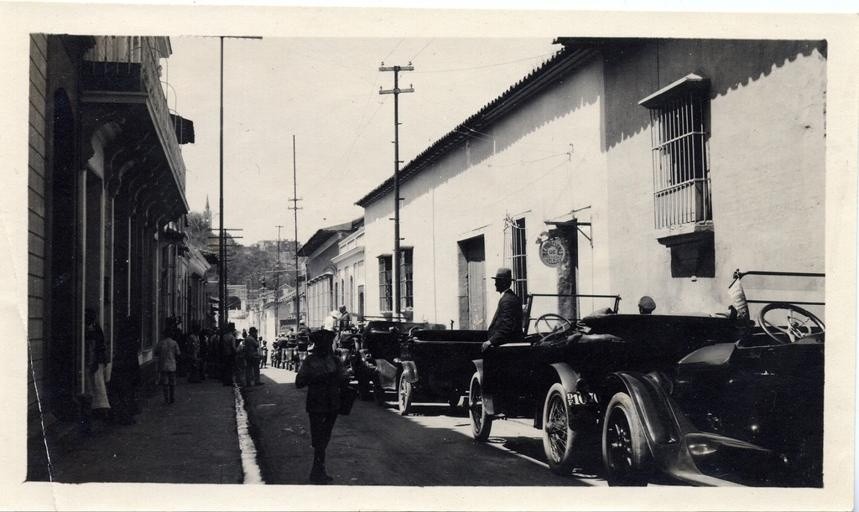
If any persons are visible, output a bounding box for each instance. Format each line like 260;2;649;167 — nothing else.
638;296;656;314
333;305;349;331
480;268;524;353
295;329;357;482
84;309;312;418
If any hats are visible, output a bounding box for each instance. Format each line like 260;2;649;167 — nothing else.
491;268;515;281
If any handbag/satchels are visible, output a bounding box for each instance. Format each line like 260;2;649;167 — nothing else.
339;385;356;415
